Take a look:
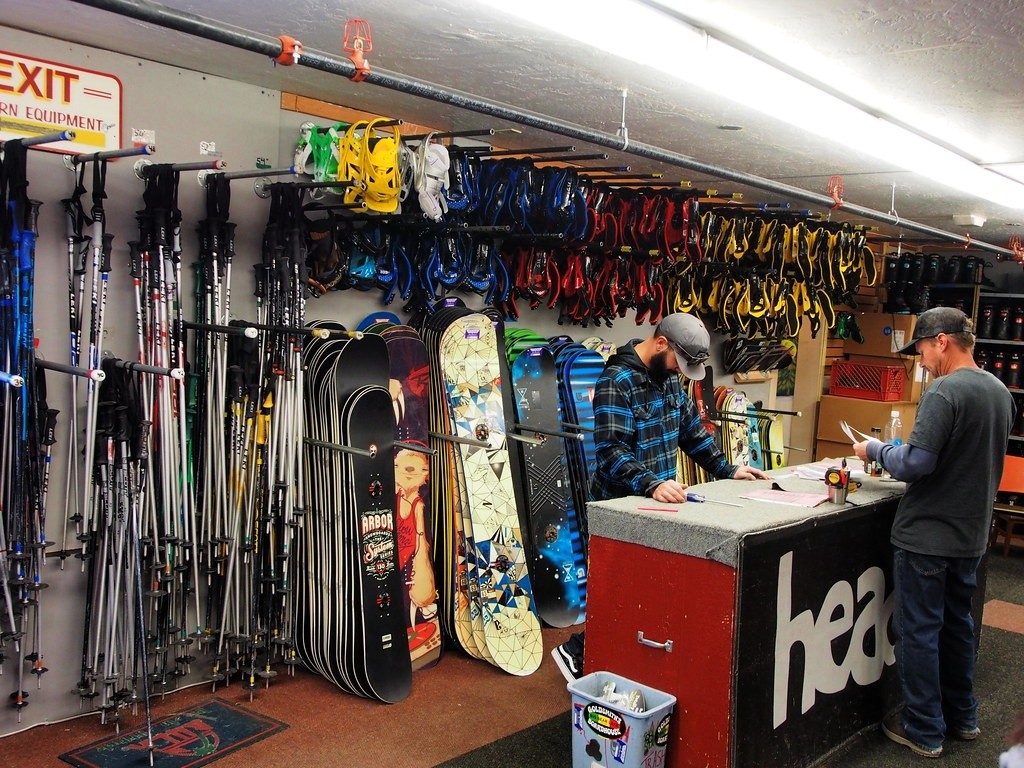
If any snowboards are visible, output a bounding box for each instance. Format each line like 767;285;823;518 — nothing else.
301;296;788;706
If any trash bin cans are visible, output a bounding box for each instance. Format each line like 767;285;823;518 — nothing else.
567;671;678;768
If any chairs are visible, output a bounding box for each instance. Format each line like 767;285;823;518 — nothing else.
987;455;1024;558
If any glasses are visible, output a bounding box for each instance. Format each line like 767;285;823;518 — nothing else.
666;337;710;366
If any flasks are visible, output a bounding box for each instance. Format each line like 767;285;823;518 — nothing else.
829;467;850;504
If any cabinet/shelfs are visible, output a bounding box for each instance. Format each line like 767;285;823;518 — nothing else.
890;264;1024;441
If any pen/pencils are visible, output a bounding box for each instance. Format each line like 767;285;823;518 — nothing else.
638;507;679;512
842;457;846;470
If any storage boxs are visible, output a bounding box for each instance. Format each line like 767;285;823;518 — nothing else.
816;312;936;462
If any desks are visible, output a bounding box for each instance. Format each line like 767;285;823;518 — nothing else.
586;456;909;768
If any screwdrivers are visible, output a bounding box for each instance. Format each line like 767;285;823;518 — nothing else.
684;492;744;508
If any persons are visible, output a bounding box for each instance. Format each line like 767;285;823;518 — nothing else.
854;306;1018;757
551;312;775;682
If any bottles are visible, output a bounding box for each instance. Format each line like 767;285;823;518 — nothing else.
884;409;903;478
865;427;883;476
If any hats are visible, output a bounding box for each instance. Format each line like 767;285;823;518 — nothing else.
896;307;973;355
660;313;711;380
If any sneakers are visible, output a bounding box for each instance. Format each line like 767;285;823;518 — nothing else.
946;724;977;739
882;720;942;757
550;642;583;682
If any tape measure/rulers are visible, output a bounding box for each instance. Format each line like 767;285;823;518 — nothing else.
823;466;844;489
847;482;858;491
